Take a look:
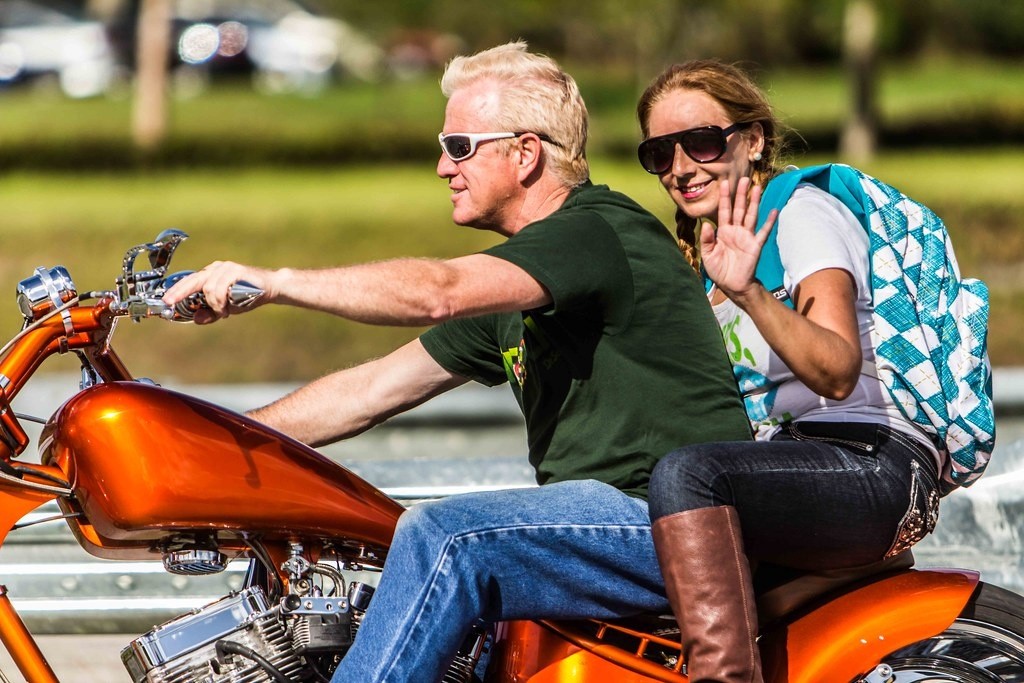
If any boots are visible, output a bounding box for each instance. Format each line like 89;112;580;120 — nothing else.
649;505;765;683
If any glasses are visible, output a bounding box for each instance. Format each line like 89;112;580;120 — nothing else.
637;122;749;175
437;130;563;162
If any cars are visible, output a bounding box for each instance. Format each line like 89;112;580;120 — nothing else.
0;0;386;105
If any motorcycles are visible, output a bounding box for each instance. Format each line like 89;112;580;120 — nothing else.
1;230;1023;683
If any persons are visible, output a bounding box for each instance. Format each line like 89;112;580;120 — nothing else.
636;58;941;683
162;40;756;683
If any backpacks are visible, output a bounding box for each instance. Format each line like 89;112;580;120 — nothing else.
748;163;997;489
